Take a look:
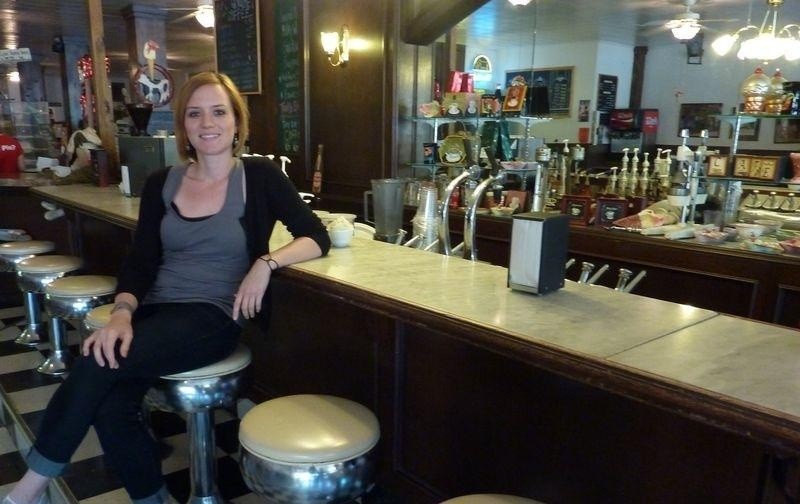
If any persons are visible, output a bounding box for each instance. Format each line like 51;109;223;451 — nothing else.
0;119;25;179
0;72;331;504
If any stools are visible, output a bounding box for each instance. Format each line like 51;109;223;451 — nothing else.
158;346;252;502
439;492;545;503
0;228;120;377
238;395;382;502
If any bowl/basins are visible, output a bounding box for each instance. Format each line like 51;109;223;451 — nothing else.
733;218;784;241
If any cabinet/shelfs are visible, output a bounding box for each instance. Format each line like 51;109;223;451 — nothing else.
706;111;799;187
407;114;545;188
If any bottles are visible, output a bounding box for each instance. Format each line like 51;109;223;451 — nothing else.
412;185;439;251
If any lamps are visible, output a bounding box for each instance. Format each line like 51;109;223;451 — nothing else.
711;0;800;62
473;54;491;83
509;1;532;8
194;0;215;30
665;0;703;40
320;25;350;68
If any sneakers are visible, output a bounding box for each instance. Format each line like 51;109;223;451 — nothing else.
0;492;48;504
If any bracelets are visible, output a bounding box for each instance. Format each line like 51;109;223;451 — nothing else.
111;301;134;314
259;252;279;273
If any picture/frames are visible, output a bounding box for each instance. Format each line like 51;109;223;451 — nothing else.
774;82;799;144
596;199;628;228
729;103;760;141
760;157;781;179
749;155;760;179
503;82;528;113
560;194;590;229
707;154;728;178
732;153;753;177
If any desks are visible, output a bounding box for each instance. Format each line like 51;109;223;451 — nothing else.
33;182;799;455
403;189;799;328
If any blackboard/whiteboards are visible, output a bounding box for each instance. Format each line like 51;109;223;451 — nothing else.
273;1;310;157
211;1;263;96
503;66;574;120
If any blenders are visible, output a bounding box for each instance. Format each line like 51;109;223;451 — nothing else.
362;178;410;243
